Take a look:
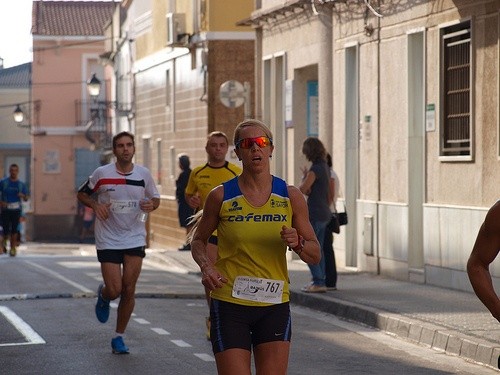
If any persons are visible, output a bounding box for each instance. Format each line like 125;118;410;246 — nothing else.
298;136;331;290
8;205;26;247
308;154;339;290
77;132;160;353
185;130;242;341
176;152;199;251
186;119;321;375
467;200;500;369
0;163;31;256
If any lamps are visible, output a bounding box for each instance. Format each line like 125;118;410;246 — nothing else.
12;105;30;128
87;72;116;108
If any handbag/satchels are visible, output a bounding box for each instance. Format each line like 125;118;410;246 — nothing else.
330;214;340;234
336;212;348;225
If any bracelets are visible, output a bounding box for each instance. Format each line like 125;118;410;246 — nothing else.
289;234;305;254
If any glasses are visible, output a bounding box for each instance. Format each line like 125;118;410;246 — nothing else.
236;136;271;149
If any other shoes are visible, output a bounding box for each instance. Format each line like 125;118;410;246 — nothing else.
10;248;16;257
300;282;327;293
178;242;192;251
325;286;337;291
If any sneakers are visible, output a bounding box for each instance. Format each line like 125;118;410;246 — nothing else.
95;284;110;324
111;336;130;354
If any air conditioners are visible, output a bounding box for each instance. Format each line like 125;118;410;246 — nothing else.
166;12;185;45
